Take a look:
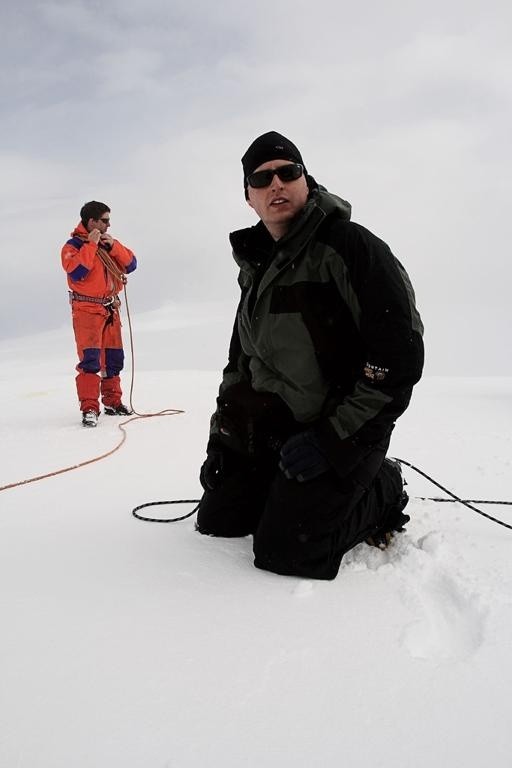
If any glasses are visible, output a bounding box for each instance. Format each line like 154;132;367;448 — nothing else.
246;164;304;189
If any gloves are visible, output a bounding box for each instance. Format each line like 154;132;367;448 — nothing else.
279;422;341;482
200;448;227;495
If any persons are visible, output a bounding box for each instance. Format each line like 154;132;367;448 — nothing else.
61;200;136;427
197;131;425;580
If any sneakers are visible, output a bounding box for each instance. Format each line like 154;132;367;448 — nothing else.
83;411;97;427
104;406;134;416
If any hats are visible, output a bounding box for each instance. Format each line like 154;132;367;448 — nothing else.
242;131;308;178
